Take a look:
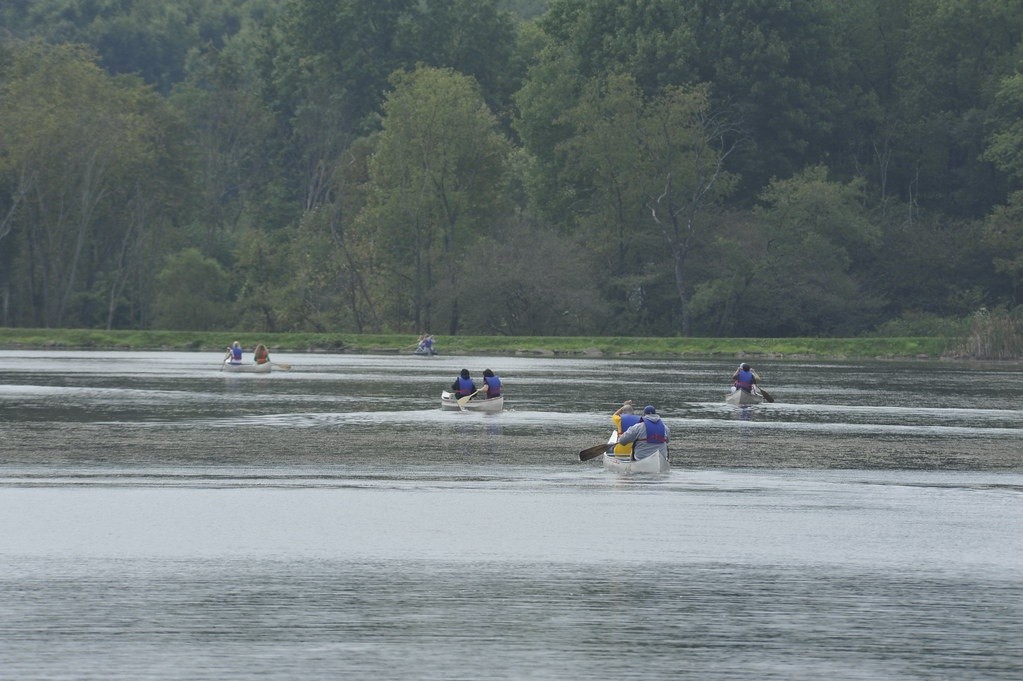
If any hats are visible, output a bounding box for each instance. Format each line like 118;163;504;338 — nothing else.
740;363;745;367
484;370;494;377
623;404;633;413
644;405;656;414
460;369;470;379
743;364;750;371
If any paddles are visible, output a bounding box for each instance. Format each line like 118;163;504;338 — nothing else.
271;362;292;369
755;384;775;403
456;391;479;406
579;442;618;462
219;349;229;371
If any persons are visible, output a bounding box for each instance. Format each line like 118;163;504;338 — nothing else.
731;363;759;395
417;334;433;352
254;344;270;364
477;369;504;399
224;341;242;361
618;406;671;461
451;369;477;399
607;400;641;458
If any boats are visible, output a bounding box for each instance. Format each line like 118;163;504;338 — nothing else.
221;357;275;376
439;389;503;416
724;387;762;406
600;427;673;477
